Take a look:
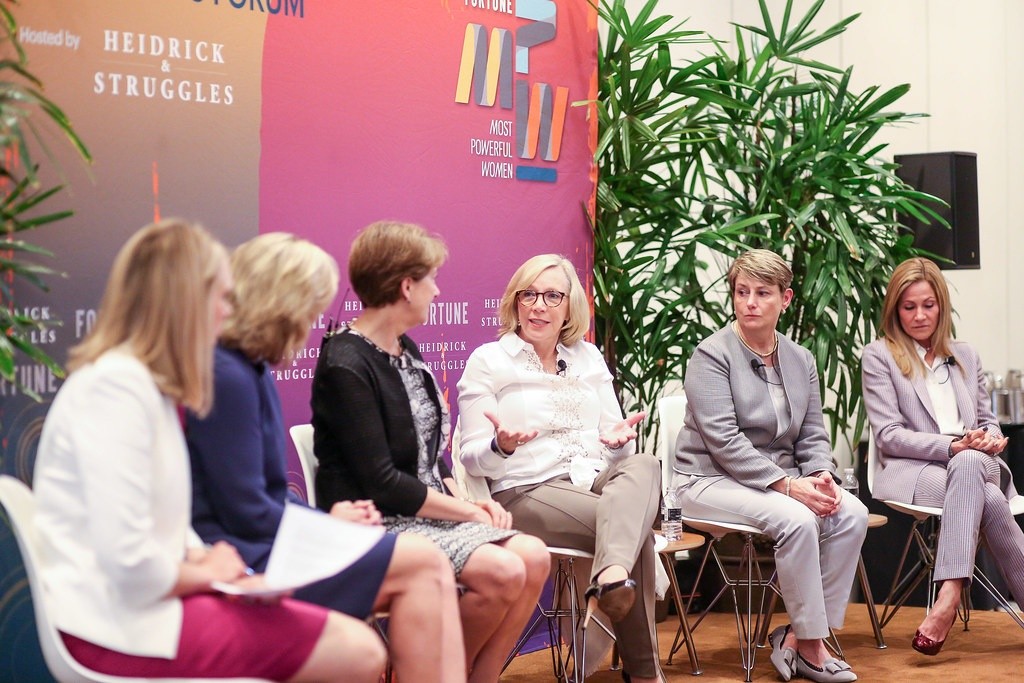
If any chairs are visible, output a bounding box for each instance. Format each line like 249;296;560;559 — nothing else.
1;398;1024;683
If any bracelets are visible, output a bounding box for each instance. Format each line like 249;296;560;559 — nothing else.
787;476;793;496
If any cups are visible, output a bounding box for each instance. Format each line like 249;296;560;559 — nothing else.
991;387;1024;424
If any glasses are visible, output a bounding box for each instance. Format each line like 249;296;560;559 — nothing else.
514;289;569;308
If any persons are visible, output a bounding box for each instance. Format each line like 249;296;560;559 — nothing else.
185;230;467;683
34;218;389;683
861;257;1024;657
457;253;667;683
672;248;869;683
309;220;552;683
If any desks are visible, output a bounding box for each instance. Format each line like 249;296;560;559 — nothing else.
757;514;889;648
857;423;1023;610
610;529;706;675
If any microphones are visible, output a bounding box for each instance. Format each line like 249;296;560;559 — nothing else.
254;362;266;377
949;356;955;366
751;359;759;371
558;359;567;371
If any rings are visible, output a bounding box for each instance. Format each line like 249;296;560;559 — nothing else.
518;441;524;445
614;445;619;449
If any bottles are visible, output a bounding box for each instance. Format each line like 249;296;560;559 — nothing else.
661;487;683;542
984;369;1024;391
840;467;860;500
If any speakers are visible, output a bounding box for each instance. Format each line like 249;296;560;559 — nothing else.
892;153;981;272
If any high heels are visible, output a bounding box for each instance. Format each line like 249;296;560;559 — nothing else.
621;670;631;683
580;578;637;630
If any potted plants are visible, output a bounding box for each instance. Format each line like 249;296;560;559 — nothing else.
568;2;957;623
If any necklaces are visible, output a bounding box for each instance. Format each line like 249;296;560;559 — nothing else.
737;320;777;357
925;347;931;351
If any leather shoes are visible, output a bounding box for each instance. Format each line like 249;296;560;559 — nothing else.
796;651;857;683
768;624;798;681
911;609;957;657
